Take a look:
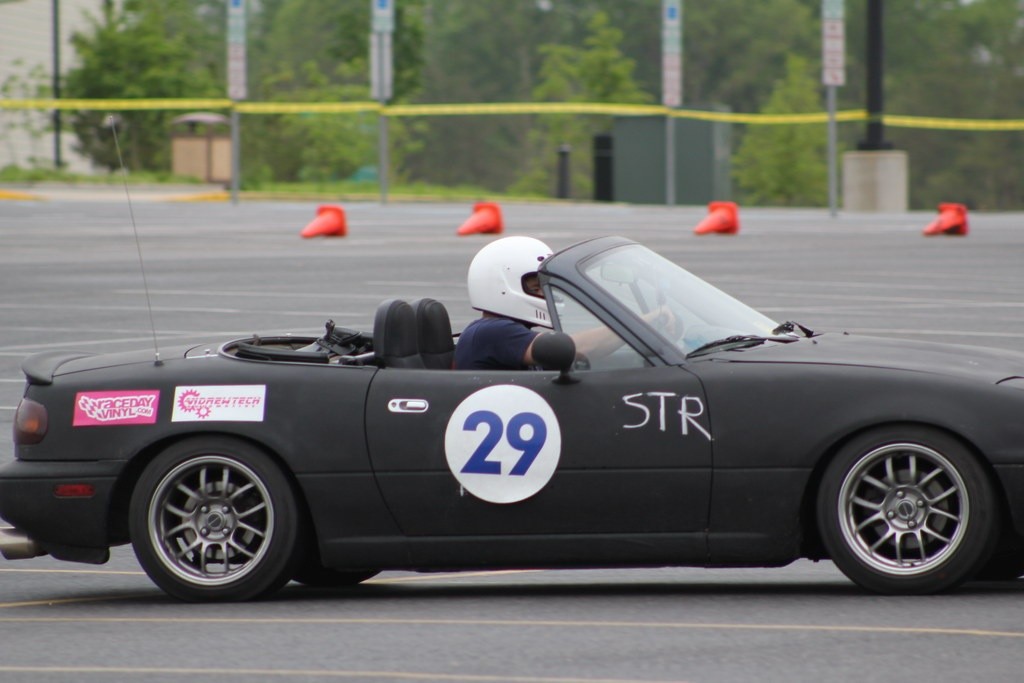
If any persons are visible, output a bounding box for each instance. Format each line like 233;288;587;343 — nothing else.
454;235;577;371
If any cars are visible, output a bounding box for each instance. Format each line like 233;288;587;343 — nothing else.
0;236;1021;598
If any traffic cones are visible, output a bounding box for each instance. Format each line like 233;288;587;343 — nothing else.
458;202;506;236
299;205;348;240
695;201;739;236
923;202;970;236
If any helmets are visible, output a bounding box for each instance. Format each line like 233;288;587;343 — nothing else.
466;235;565;329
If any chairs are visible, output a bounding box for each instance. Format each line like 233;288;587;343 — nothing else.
373;295;455;371
